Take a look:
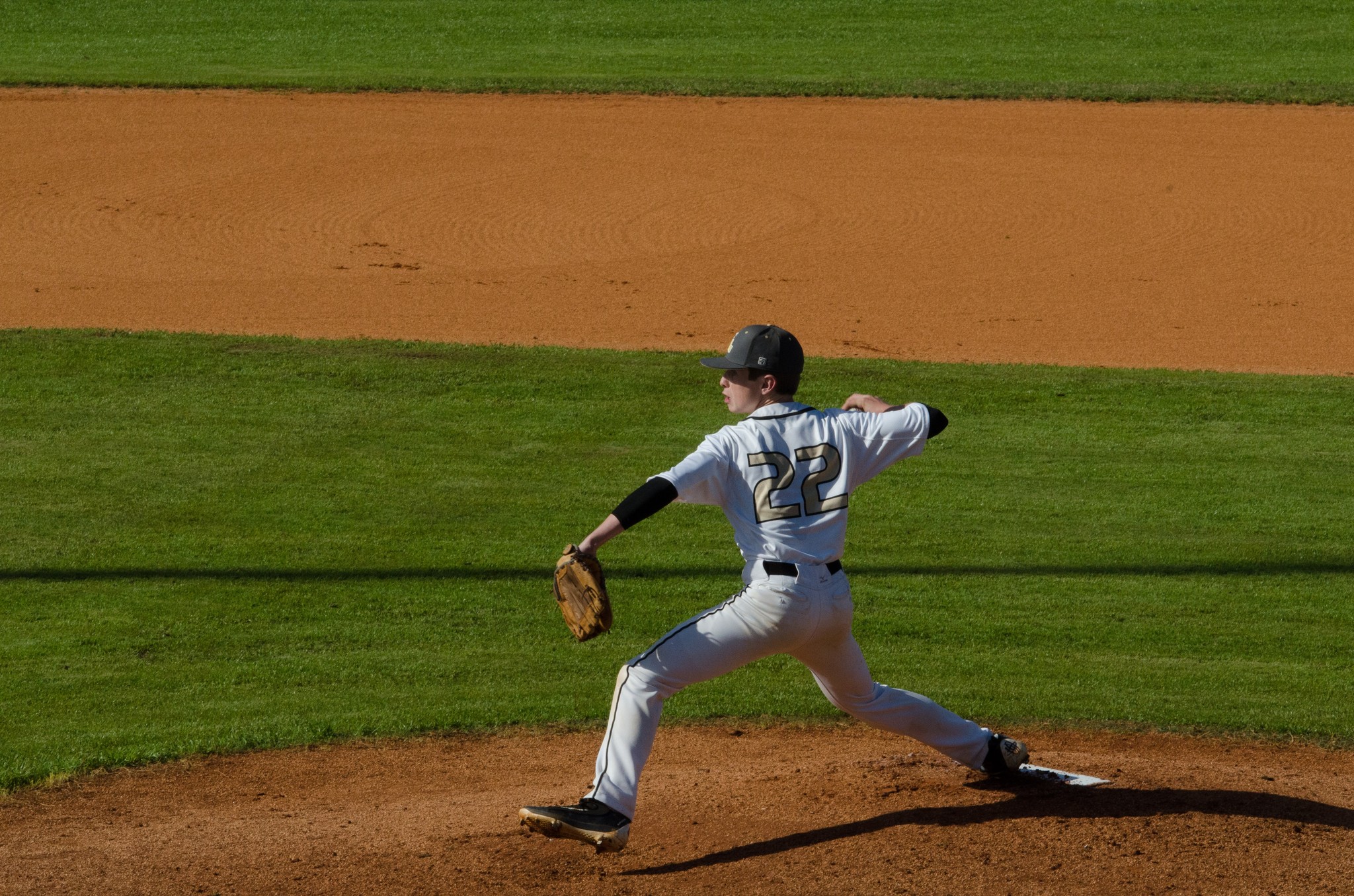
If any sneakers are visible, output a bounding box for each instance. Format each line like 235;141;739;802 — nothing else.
518;797;631;855
979;733;1032;784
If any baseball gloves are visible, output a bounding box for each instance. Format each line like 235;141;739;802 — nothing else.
552;544;613;643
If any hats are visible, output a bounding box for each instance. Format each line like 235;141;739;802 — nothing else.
700;323;805;376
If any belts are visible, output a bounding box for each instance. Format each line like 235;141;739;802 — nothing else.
762;556;842;577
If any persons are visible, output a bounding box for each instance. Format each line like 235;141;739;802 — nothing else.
517;323;1031;851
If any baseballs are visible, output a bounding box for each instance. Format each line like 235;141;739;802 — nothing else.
844;405;865;413
